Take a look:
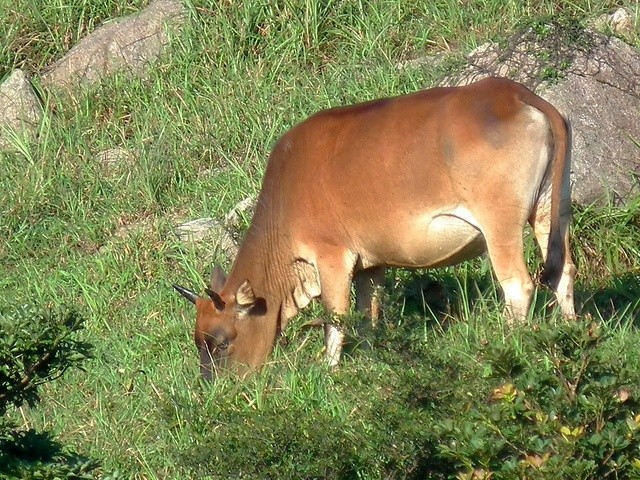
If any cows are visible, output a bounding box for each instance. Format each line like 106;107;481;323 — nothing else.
170;76;577;386
